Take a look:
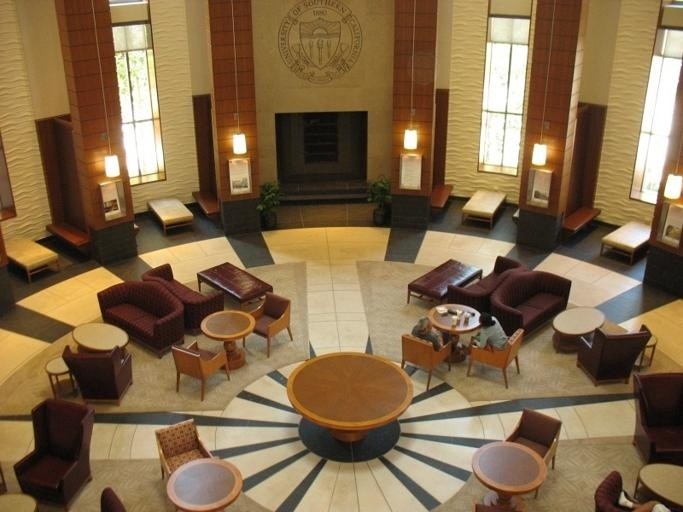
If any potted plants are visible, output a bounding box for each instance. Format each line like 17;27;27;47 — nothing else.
256;180;285;227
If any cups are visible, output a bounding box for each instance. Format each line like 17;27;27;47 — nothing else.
451;309;470;327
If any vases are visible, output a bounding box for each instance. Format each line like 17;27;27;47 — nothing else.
367;175;392;225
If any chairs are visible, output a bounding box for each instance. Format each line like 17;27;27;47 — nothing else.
505;406;563;500
466;328;525;392
100;487;126;510
246;292;295;357
575;323;654;386
631;371;682;466
474;502;517;510
156;418;214;478
401;334;453;390
61;341;134;407
14;398;95;510
171;341;230;402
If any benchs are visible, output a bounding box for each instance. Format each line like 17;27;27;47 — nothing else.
431;184;453;218
134;223;140;237
513;209;520;227
563;208;601;237
46;222;95;257
193;191;220;226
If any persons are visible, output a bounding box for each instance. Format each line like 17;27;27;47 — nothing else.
462;313;507;354
412;317;454;352
619;491;673;512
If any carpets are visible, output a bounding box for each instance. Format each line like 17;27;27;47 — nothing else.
49;253;72;272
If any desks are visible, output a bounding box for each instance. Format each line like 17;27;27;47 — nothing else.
0;494;36;512
461;189;507;229
148;198;195;235
73;323;130;353
632;463;683;511
165;457;244;511
286;351;414;443
472;440;548;510
429;303;482;364
600;220;652;264
552;306;605;354
634;335;657;370
200;310;255;370
4;236;59;284
44;357;79;401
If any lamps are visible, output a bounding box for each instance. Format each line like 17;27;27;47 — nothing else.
664;133;682;200
91;0;120;177
531;0;556;166
231;0;247;155
404;0;418;150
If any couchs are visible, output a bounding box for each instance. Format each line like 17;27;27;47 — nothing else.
141;263;224;336
447;255;521;324
593;470;643;511
98;280;185;358
490;269;573;334
